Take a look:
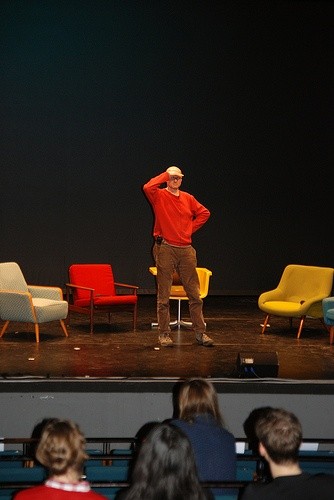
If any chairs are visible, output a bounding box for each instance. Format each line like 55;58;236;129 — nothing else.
0;262;69;343
62;264;139;334
258;264;334;346
148;266;212;328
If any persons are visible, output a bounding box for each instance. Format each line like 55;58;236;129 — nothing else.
240;408;334;500
169;378;237;481
115;419;208;500
13;418;108;500
142;166;215;347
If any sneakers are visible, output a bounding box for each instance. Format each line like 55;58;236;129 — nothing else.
196;333;214;346
157;335;175;346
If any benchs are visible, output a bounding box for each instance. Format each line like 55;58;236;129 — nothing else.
0;434;334;500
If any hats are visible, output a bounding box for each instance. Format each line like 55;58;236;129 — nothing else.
166;167;185;176
